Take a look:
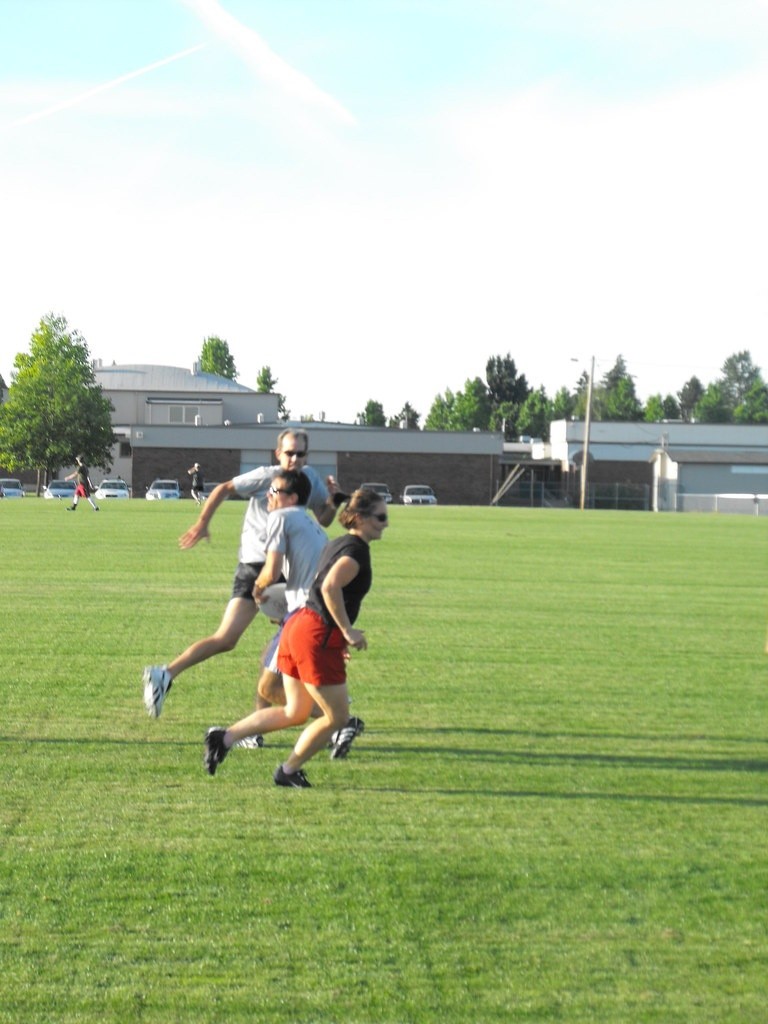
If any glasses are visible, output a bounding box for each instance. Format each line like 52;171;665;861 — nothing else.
370;513;387;521
269;486;291;499
284;451;305;457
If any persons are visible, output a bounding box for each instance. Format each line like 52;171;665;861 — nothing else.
64;454;97;510
206;487;388;787
235;470;366;760
143;429;343;748
189;462;207;505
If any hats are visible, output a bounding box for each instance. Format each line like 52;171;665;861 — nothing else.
76;455;84;462
194;463;200;468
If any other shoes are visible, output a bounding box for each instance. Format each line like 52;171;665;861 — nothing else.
67;506;76;511
94;506;99;512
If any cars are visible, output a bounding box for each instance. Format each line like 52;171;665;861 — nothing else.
41;479;77;500
399;484;437;506
145;479;183;500
355;482;395;505
0;478;26;498
93;479;129;500
198;482;231;501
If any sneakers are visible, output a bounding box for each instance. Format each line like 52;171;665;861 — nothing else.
275;765;312;788
142;664;176;719
331;717;364;759
204;727;232;775
235;734;263;748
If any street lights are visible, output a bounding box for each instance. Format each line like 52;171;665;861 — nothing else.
569;357;596;509
